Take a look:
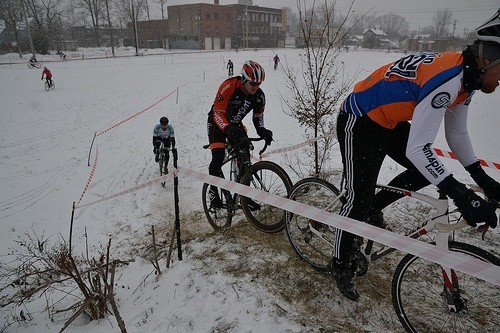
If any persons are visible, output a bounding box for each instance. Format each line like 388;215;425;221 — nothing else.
208;60;273;212
58;51;68;62
226;60;233;76
41;66;53;88
327;8;500;301
153;117;176;174
28;54;38;67
273;54;280;70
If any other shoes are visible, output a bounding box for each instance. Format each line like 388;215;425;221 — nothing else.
155;155;159;162
165;168;168;174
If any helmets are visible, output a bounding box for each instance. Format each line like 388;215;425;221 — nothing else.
160;117;168;125
241;60;265;82
475;9;500;43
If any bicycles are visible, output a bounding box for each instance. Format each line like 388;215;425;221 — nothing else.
284;166;500;333
41;78;55;91
202;132;295;233
152;143;173;187
28;60;41;69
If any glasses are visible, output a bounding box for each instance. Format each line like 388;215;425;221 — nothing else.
246;80;261;86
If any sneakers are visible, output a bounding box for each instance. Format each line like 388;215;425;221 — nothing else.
209;189;222;208
363;205;386;229
327;257;360;301
246;199;260;211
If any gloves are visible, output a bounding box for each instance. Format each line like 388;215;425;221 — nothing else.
223;124;242;143
437;175;498;228
257;127;273;145
464;161;500;202
